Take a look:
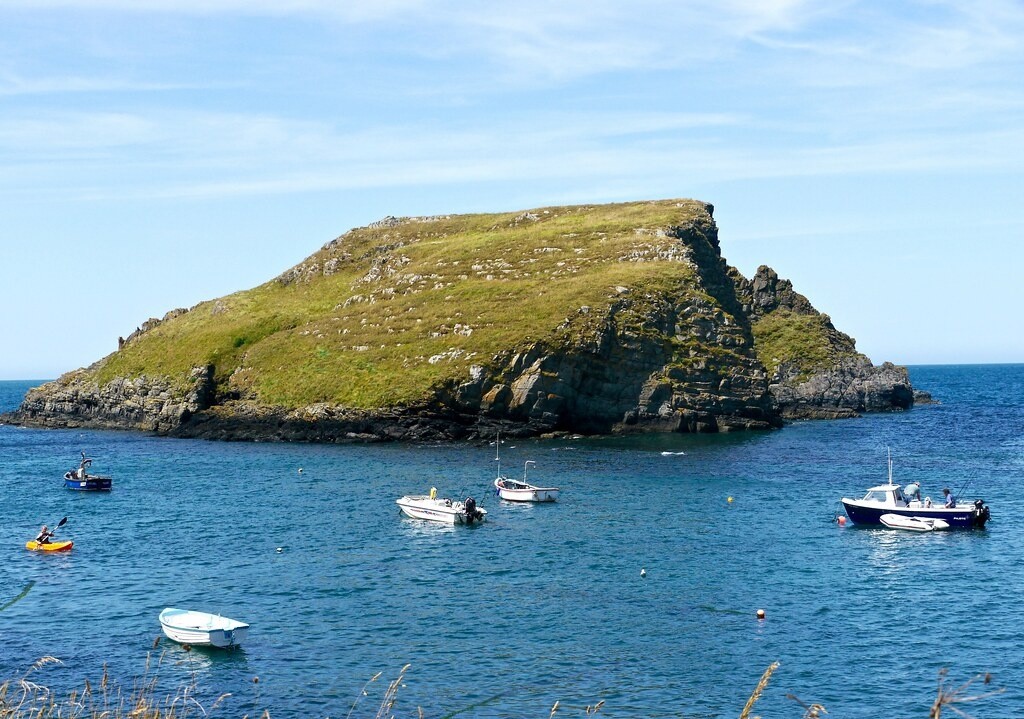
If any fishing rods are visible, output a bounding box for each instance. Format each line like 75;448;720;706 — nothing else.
955;463;978;500
478;479;493;509
455;474;470;508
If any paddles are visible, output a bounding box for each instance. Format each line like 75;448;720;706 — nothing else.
39;517;68;544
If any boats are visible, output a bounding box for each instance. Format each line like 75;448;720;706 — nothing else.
395;494;488;527
63;452;113;492
493;460;561;502
25;540;74;552
158;607;250;648
841;446;991;532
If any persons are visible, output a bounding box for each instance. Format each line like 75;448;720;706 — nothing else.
430;486;438;501
35;525;54;545
904;481;920;507
942;488;957;509
